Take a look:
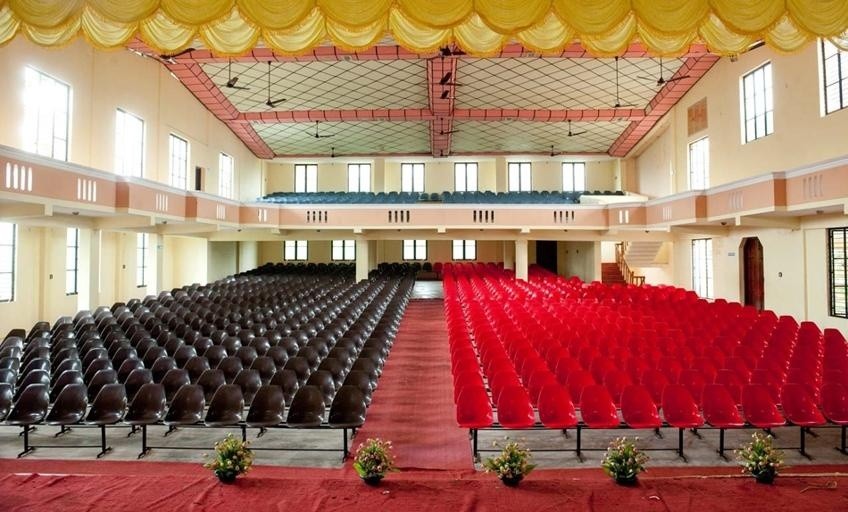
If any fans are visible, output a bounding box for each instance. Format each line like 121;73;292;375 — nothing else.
146;48;345;160
424;45;467;159
544;40;768;158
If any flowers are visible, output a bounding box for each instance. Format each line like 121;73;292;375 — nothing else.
478;435;538;480
199;433;255;478
598;436;647;479
350;438;400;479
733;433;785;473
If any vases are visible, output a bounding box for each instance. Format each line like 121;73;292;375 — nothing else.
751;471;774;485
502;475;521;486
365;475;383;487
616;475;636;485
216;471;237;485
